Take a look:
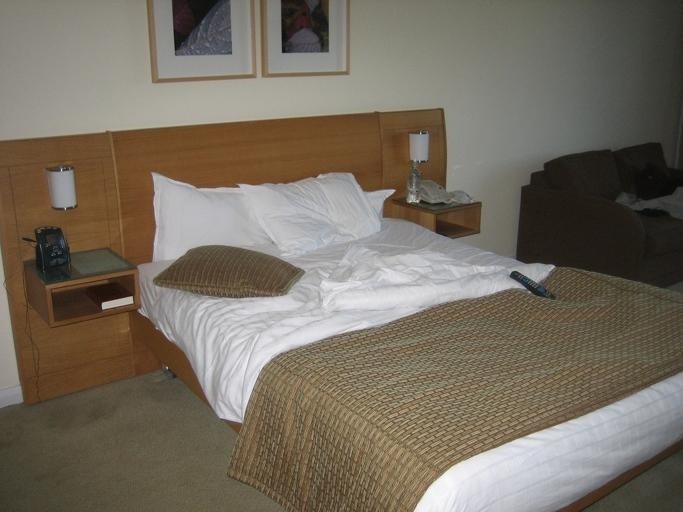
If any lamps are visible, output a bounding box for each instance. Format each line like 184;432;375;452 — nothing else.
47;163;78;212
409;129;429;165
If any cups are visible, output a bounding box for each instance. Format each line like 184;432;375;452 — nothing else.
406;174;424;205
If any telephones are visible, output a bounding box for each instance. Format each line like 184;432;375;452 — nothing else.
419;179;448;205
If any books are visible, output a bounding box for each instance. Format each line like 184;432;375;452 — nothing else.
88;285;134;312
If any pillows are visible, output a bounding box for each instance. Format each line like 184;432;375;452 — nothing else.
614;143;671;195
545;149;623;200
148;172;280;260
154;244;304;300
239;172;382;251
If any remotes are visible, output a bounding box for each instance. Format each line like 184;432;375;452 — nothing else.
509;270;554;299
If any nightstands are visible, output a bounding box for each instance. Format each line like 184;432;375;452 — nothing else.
16;247;141;327
393;186;482;239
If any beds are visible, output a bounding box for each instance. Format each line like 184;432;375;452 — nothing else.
0;109;683;511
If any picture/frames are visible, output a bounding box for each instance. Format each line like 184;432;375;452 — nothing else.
260;0;351;78
147;0;257;83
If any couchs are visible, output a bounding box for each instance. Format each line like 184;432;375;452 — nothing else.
516;168;683;289
